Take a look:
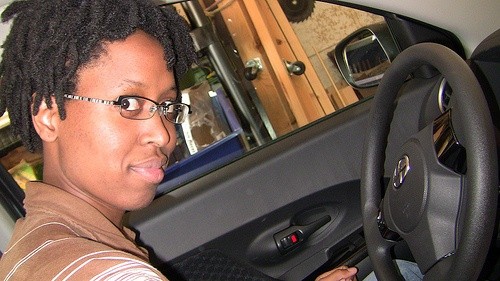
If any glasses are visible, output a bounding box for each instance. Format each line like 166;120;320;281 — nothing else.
51;93;193;124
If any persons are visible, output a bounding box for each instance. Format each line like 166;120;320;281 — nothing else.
0;0;360;281
